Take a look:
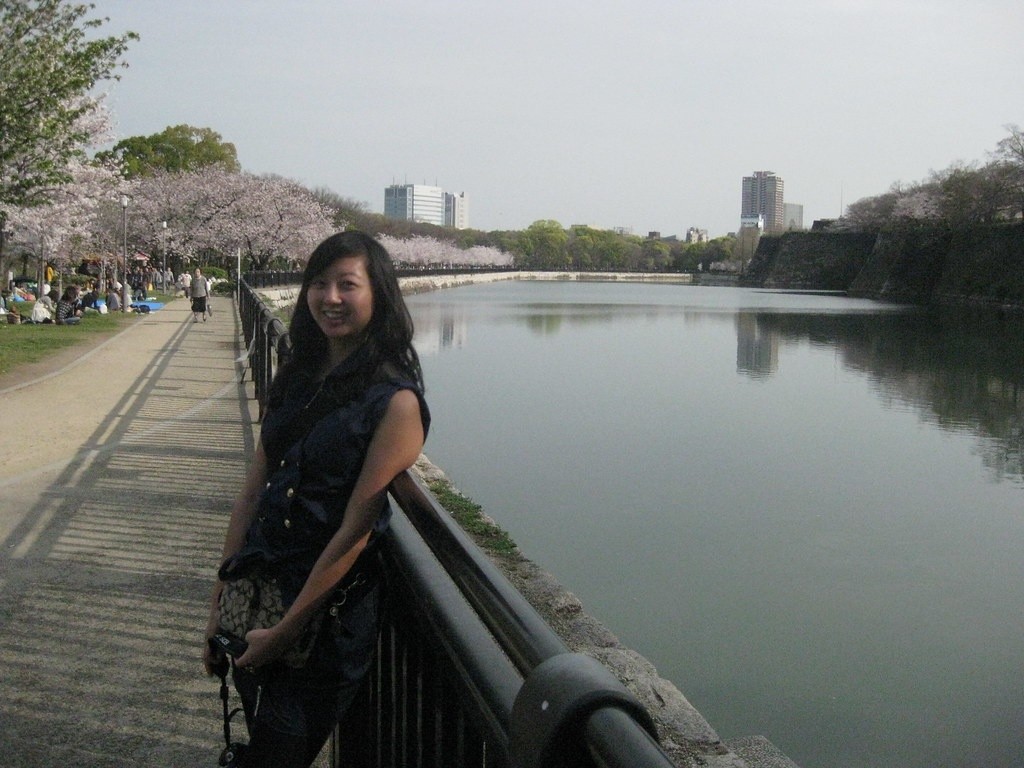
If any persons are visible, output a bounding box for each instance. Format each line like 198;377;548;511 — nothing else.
181;270;192;298
103;261;164;311
201;230;432;767
189;267;210;323
0;278;51;314
81;291;99;314
55;286;80;325
165;267;174;291
30;290;59;323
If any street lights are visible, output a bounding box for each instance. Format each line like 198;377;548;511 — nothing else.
121;195;131;312
161;222;167;294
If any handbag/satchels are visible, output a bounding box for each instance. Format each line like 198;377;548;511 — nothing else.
207;298;213;318
208;548;348;679
